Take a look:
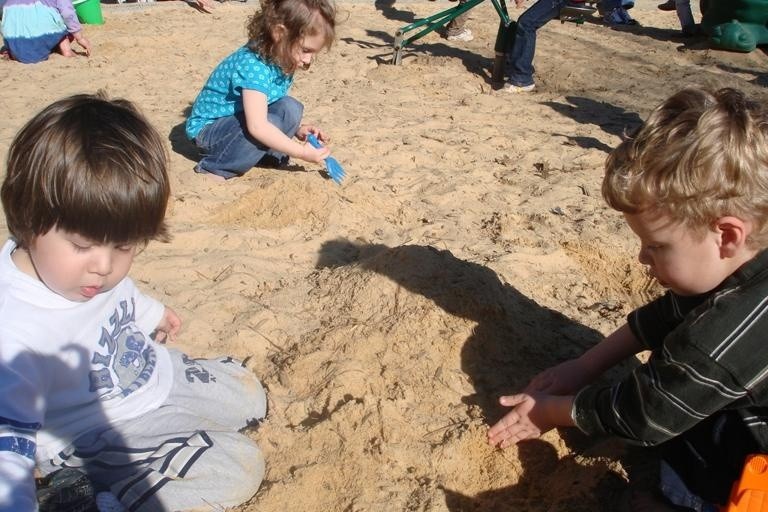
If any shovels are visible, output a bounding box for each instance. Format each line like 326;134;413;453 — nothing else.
307;134;348;184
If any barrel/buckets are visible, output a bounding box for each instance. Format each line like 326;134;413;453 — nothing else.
72;0;106;26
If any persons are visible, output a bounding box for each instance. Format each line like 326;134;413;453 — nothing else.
502;0;573;93
445;0;474;42
0;89;269;512
0;0;92;64
485;88;768;510
194;0;216;15
657;0;690;12
185;0;338;179
595;0;639;26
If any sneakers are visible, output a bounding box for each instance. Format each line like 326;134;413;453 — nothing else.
658;0;676;10
598;0;640;28
447;29;473;42
493;67;535;95
36;466;106;512
